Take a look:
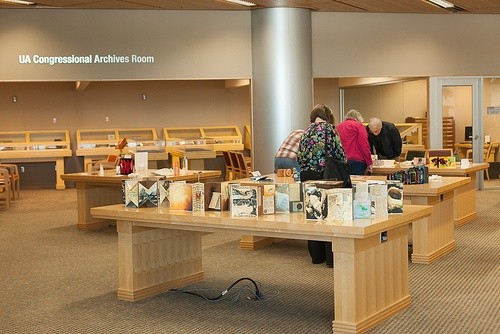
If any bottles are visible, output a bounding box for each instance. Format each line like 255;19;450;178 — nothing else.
173;162;180;176
181;156;188;172
115;154;133;175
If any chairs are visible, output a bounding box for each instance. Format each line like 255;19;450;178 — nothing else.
0;164;20;207
404;149;427;164
427;148;453;158
222;150;251;181
483;145;492;180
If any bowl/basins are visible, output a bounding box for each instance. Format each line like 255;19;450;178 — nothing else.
381;160;395;168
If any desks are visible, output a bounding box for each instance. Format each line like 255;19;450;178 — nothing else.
372;160;489;227
60;169;222;231
223;176;472;264
90;203;435;334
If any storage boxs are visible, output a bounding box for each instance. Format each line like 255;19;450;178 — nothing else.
127;135;214;154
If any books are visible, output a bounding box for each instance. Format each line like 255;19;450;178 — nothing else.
229;180;403;221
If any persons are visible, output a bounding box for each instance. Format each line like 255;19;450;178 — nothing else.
306;189;326;219
335;110;373;175
274;130;308;174
297;103;349;267
365;118;403;162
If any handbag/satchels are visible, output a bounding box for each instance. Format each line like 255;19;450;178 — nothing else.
322;157;352;188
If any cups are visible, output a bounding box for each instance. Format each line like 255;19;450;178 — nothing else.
460;158;470;169
370;155;378;166
